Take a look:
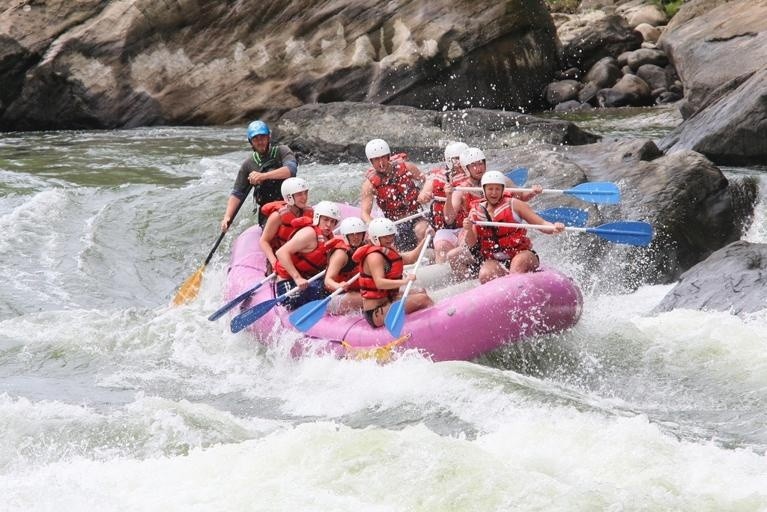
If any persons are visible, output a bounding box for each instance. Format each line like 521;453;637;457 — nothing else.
220;120;298;235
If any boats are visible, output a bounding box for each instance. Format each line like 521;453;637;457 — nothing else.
224;199;582;365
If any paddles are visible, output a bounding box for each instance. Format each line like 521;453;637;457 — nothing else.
287;274;362;331
446;182;619;205
393;168;527;224
467;221;654;246
385;233;433;339
172;159;275;305
431;191;588;227
208;271;274;322
229;267;326;333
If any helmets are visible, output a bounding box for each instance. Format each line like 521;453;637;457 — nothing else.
281;176;309;210
247;120;272;147
444;140;506;198
310;199;398;253
363;138;393;169
